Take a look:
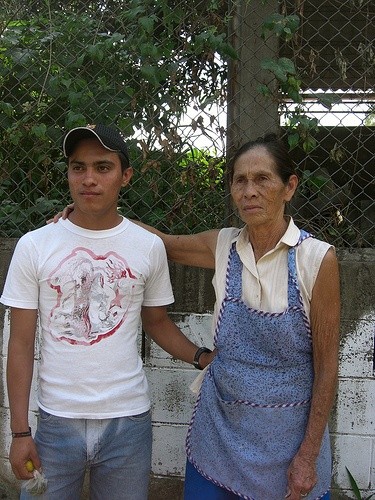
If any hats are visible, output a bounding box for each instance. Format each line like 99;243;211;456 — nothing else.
62;123;130;161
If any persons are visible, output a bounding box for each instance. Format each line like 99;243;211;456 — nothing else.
2;121;216;500
46;135;343;500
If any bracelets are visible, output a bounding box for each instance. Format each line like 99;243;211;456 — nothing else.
11;426;32;438
192;347;213;371
